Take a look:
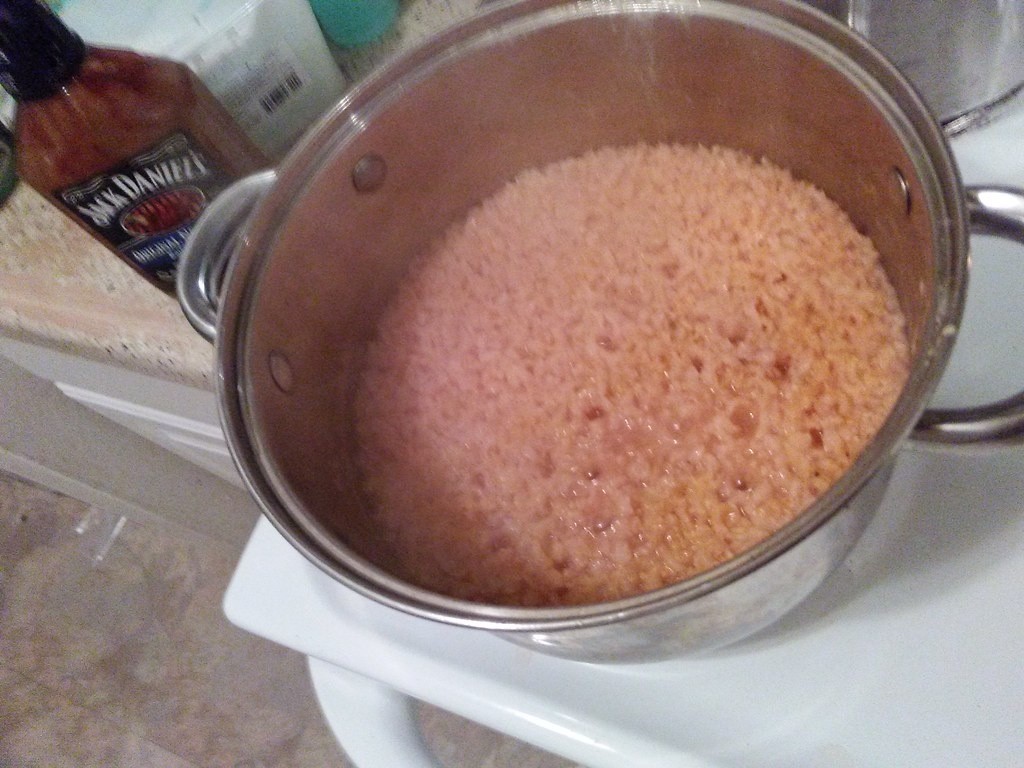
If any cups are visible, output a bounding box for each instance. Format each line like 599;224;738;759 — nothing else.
801;1;1023;124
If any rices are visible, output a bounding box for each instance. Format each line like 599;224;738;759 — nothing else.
355;137;906;607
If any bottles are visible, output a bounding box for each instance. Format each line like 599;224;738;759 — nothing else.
0;0;276;296
309;1;400;48
56;0;350;160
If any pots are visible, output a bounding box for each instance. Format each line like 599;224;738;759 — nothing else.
175;1;1023;669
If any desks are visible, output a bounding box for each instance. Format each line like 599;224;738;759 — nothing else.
220;108;1024;768
